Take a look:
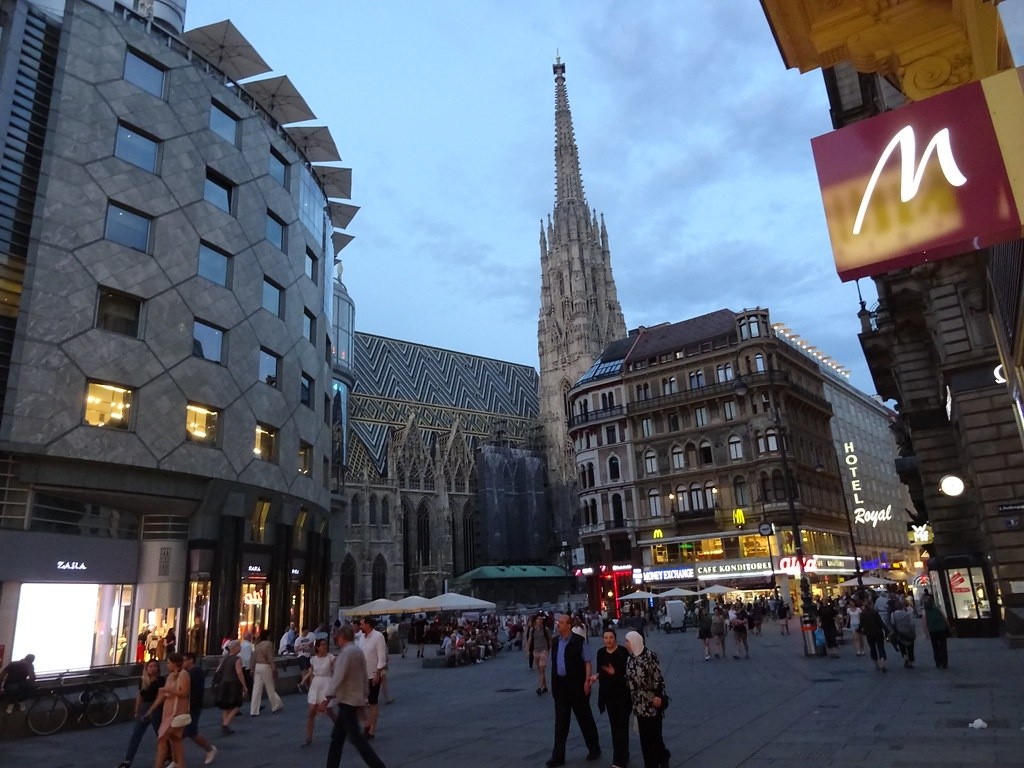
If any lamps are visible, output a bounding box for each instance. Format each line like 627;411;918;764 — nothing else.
712;487;719;507
669;494;676;515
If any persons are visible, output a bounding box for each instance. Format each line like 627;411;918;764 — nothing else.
298;637;337;748
120;658;167;768
215;640;248;735
137;628;176;662
236;633;265;717
588;627;635;767
546;614;601;768
278;614;525;696
624;631;671;768
250;631;284;715
525;597;796;696
814;588;951;670
144;654;190;768
358;617;387;741
0;655;37;714
182;652;218;768
322;625;387;767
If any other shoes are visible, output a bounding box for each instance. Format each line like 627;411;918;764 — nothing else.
451;655;495;667
218;705;284;737
545;757;566;768
204;745;217;763
301;738;314;748
385;697;394;704
297;683;303;693
365;733;375;741
363;726;370;734
832;649;949;672
117;761;134;768
506;647;522;652
542;687;549;693
536;687;541;695
586;749;603;761
528;667;533;672
166;761;176;768
702;651;748;661
750;627;794;638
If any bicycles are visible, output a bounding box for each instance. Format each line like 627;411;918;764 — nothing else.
25;669;132;736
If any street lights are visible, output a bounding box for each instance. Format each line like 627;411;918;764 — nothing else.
815;440;863;589
558;539;572;611
734;342;823;657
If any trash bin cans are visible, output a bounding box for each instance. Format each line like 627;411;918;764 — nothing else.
799;612;822;657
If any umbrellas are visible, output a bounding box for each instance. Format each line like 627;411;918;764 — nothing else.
657;587;703;597
344;599;395;616
388;593;496;614
697;584;737;596
617;590;657;599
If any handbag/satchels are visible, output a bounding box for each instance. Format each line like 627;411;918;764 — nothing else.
653;694;668;710
213;671;224;684
815;627;826;646
171;714;191;727
